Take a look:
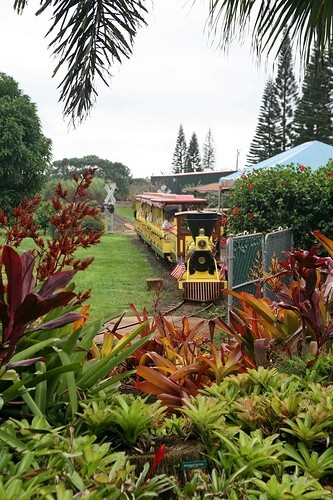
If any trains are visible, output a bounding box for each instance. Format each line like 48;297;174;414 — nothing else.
133;192;228;301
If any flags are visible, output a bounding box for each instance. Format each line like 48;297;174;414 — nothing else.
218;261;228;281
170;256;187;281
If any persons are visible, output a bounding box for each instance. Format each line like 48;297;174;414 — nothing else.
160;212;177;236
